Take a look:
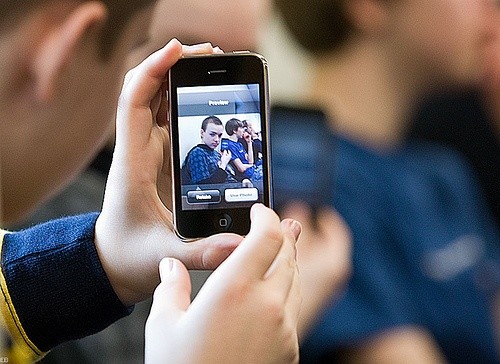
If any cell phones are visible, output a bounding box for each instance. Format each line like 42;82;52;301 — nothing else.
165;51;272;242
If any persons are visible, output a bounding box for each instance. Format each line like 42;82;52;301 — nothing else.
182;116;252;184
0;0;304;364
264;1;500;363
238;119;262;165
225;118;262;180
